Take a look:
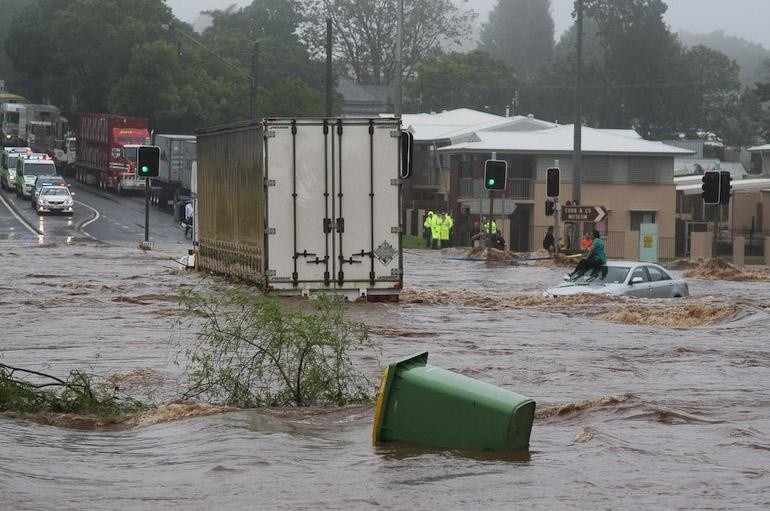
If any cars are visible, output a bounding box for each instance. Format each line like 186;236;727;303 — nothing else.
541;260;689;300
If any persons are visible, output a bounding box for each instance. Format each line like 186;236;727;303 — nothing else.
485;218;497;235
543;226;562;250
182;200;194;241
439;210;453;248
423;211;442;249
582;234;594;253
565;231;607;275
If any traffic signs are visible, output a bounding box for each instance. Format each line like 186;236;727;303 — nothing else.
561;204;610;223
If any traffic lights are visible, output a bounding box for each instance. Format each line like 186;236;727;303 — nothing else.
544;200;555;216
702;170;720;203
720;171;733;205
137;145;159;177
485;159;508;191
546;167;560;199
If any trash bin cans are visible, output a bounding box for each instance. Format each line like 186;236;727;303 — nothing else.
370;349;537;451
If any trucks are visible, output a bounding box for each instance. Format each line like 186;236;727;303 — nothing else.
73;111;151;194
179;115;405;304
148;132;198;222
0;103;77;215
